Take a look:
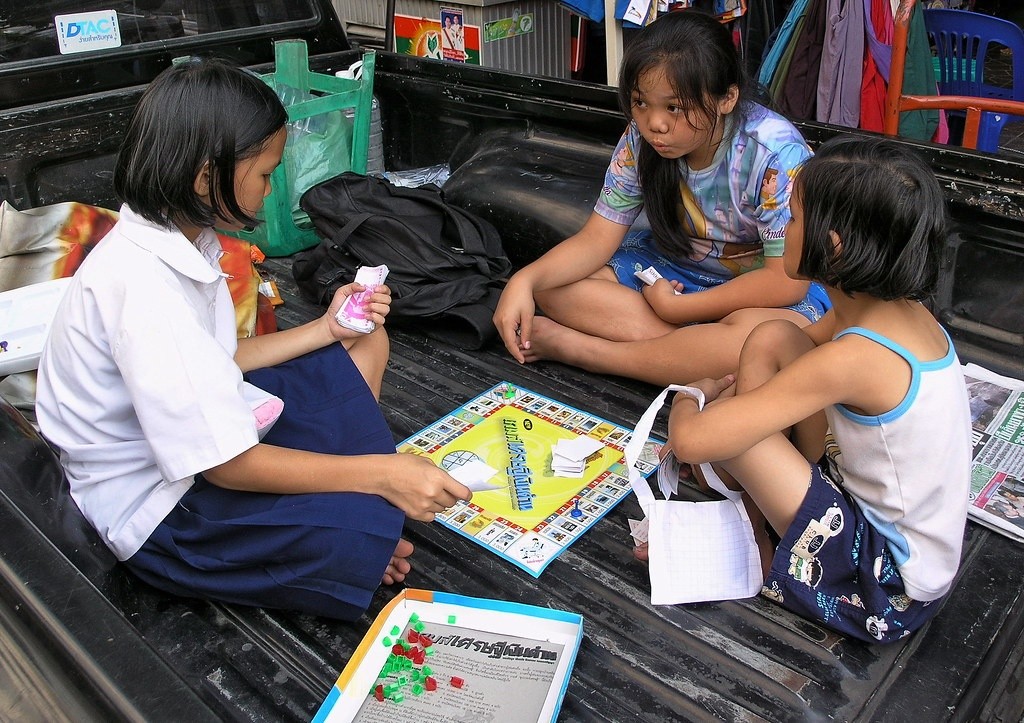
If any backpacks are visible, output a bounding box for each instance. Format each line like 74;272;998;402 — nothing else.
292;172;512;350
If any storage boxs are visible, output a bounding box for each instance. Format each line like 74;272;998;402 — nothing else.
311;589;584;723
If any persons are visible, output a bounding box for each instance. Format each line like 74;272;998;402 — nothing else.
993;490;1024;530
634;137;973;644
34;58;472;609
442;15;463;51
493;8;832;386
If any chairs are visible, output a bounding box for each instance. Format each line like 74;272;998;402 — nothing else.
923;8;1024;154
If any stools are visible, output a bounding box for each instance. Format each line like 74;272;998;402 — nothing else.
171;38;376;257
